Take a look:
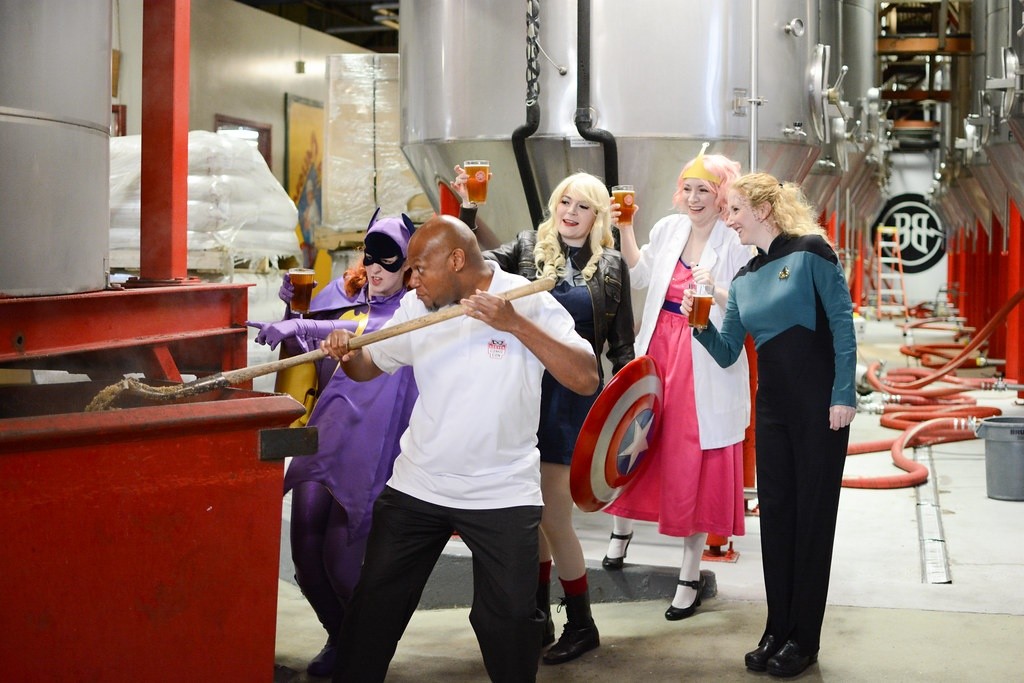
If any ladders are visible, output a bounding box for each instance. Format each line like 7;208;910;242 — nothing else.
869;226;907;323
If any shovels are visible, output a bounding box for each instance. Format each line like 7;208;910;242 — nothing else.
114;277;555;400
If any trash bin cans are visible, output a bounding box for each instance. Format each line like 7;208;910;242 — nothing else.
979;416;1023;501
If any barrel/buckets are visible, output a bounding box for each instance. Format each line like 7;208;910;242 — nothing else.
975;415;1024;503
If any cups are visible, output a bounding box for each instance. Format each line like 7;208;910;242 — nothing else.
688;283;715;329
463;160;490;203
611;184;635;225
289;267;315;315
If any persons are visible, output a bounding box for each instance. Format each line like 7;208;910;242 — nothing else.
481;166;635;665
244;213;598;682
680;170;857;677
602;155;759;617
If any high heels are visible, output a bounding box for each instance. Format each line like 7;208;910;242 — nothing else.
602;529;634;569
665;571;705;620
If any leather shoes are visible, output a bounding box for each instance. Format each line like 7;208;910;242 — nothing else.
766;640;818;677
744;634;787;672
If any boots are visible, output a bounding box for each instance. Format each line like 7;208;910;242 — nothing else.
542;584;600;665
540;584;555;646
